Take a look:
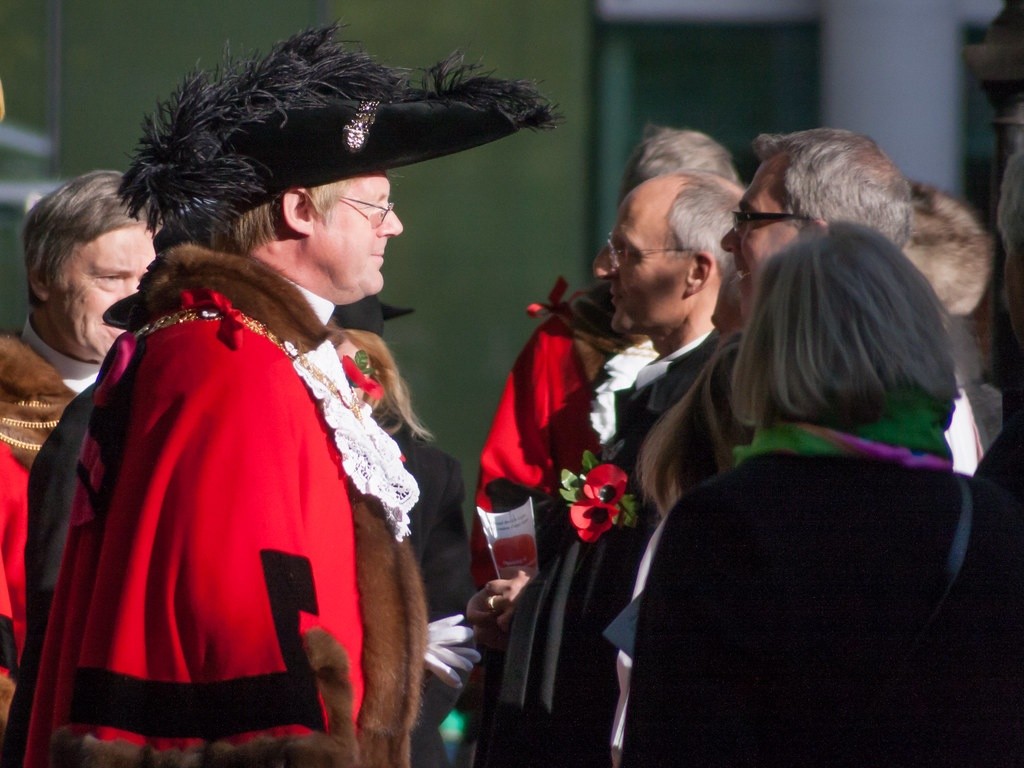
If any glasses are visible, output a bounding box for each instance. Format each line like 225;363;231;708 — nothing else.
607;230;693;270
732;209;810;238
339;192;396;226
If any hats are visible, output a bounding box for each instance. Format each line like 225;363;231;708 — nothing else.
116;15;568;241
331;295;415;338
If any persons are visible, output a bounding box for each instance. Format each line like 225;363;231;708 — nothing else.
0;20;1024;767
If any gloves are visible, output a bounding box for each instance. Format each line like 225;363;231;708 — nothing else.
425;614;481;690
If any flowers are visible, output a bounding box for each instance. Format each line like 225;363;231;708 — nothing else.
559;450;640;541
